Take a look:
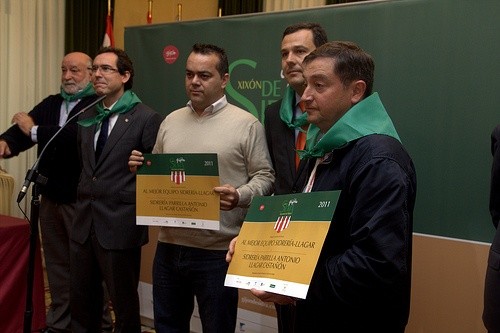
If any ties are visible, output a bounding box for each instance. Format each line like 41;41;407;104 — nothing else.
95;109;113;166
295;101;310;175
303;150;332;193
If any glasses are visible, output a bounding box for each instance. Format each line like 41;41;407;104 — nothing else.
87;65;119;73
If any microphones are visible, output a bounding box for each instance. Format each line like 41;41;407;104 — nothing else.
17;95;107;203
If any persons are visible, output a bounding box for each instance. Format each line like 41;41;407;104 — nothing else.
0;51;114;333
264;22;329;333
128;42;275;333
225;41;417;333
481;123;500;333
42;46;162;333
